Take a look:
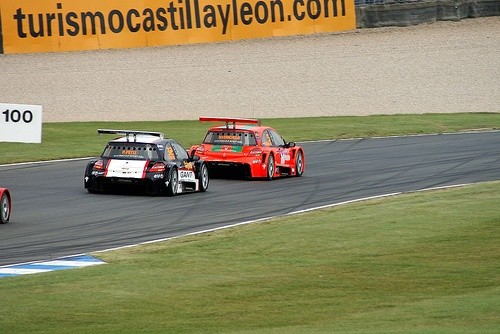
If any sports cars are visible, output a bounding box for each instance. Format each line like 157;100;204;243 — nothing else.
84;128;209;196
188;116;305;180
